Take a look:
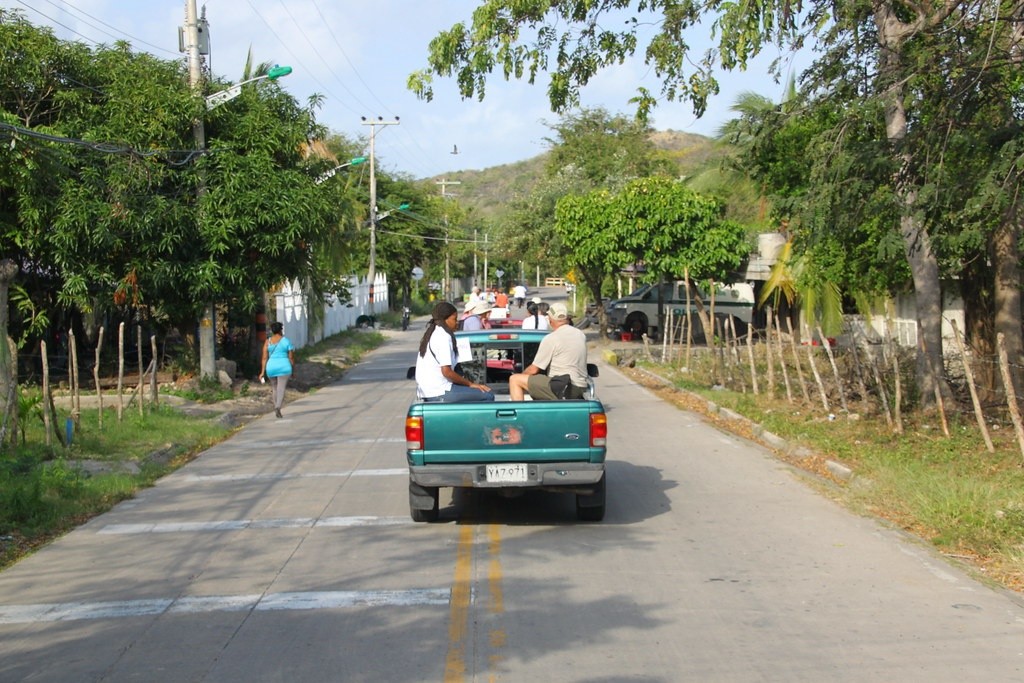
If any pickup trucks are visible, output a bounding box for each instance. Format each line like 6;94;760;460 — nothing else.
403;329;609;522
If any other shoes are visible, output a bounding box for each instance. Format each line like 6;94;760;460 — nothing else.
274;408;282;419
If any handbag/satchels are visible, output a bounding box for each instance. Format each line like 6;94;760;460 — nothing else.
549;374;572;400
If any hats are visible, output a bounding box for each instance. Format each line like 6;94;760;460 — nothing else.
463;299;477;313
472;286;480;290
472;300;492;315
546;303;568;320
532;297;542;305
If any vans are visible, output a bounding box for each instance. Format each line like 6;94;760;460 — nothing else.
604;279;757;340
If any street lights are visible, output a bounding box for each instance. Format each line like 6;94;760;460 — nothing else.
190;64;293;384
304;157;370;349
366;204;410;326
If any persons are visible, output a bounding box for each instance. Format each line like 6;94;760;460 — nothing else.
259;322;295;418
522;297;550;329
513;284;527;305
415;302;494;402
461;287;509;331
509;303;588;401
